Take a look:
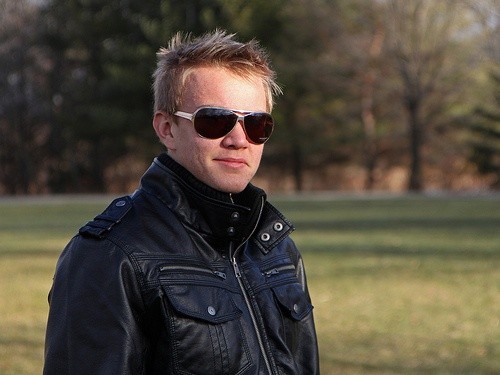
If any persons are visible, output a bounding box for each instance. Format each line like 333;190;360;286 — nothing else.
41;25;321;374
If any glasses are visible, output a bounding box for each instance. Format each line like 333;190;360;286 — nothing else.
172;105;275;146
